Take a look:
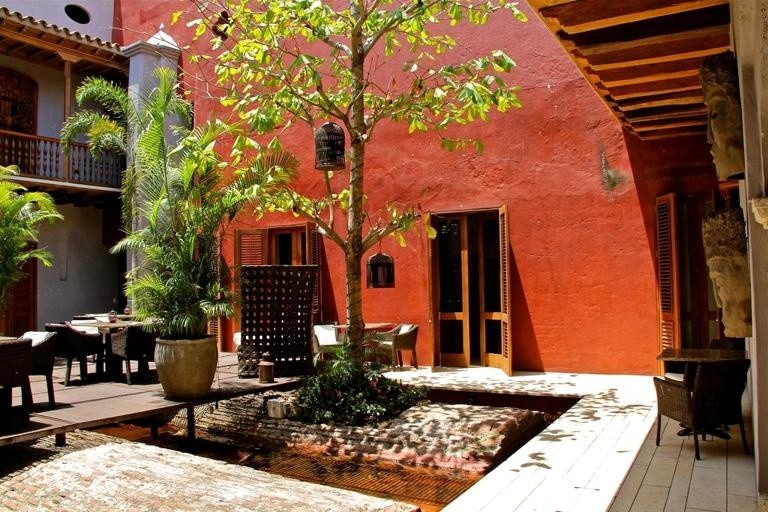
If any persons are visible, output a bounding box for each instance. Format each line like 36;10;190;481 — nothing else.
700;49;750;185
699;207;754;340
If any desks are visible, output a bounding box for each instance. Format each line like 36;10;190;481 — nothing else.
656;349;748;440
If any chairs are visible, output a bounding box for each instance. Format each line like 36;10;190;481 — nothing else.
653;359;751;459
709;339;745;350
313;321;419;370
0;314;155;412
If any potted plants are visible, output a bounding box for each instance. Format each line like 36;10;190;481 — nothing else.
59;66;299;401
